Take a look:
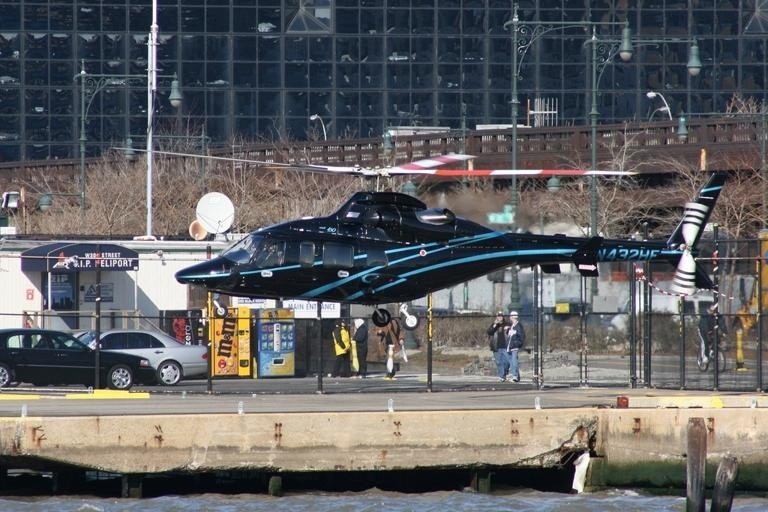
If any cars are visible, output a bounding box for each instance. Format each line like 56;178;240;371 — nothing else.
1;324;210;393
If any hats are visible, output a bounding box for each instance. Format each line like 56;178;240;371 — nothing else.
496;310;504;318
509;310;518;319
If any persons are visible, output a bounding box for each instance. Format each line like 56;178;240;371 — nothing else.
350;319;368;379
487;311;511;383
331;318;351;377
506;312;525;381
256;243;279;265
377;318;405;378
697;303;728;363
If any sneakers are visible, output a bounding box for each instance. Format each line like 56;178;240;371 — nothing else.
497;376;519;382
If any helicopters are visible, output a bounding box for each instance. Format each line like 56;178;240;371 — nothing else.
106;134;728;332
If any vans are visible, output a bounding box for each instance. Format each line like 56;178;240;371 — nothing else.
606;291;718;349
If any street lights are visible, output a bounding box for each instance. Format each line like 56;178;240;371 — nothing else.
31;61;183;234
502;1;634;314
645;90;674;135
122;127;215;203
581;24;706;314
310;112;328;144
643;107;669;137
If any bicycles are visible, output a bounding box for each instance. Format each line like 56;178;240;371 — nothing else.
696;328;729;374
